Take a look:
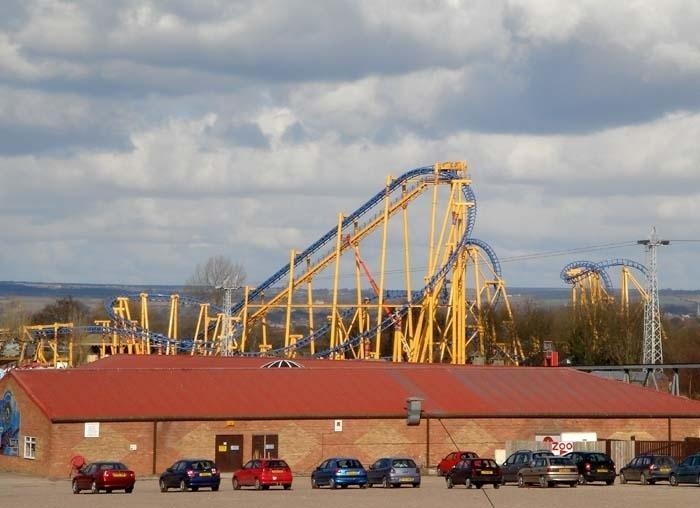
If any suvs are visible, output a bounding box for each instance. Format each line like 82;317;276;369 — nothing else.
500;448;555;483
619;454;677;485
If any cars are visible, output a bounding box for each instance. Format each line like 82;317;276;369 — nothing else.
515;456;580;490
561;450;618;481
366;456;422;489
230;459;294;491
436;451;482;477
159;459;221;493
310;457;369;490
71;461;136;495
668;453;700;487
444;458;504;490
